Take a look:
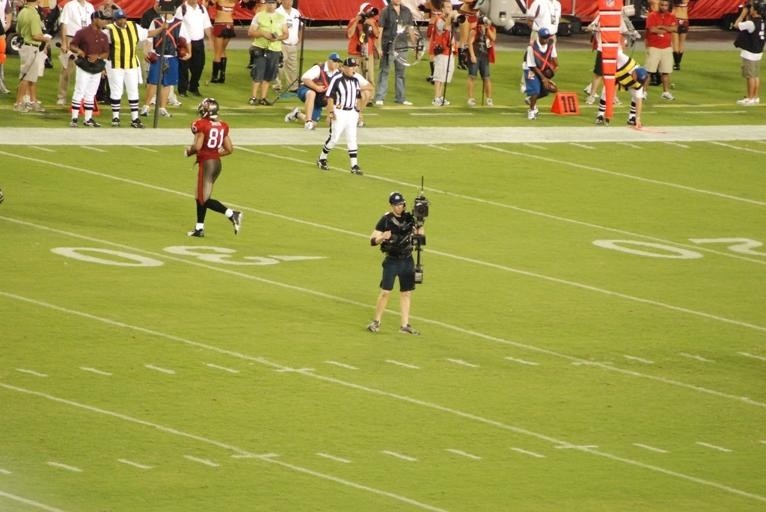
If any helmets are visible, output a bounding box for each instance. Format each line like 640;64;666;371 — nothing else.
539;29;550;38
199;98;219;119
636;68;648;83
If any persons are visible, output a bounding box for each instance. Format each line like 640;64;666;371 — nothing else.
368;192;424;336
186;98;242;237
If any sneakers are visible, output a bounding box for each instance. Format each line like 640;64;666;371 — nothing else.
84;118;102;127
367;320;382;333
305;122;315;129
432;96;493;109
404;100;412;105
736;96;759;104
375;100;384;105
111;118;119;127
0;89;46;114
317;159;328;169
150;91;201;106
70;117;78;127
56;98;67;104
661;92;675;100
140;105;149;117
159;108;172;117
187;229;204;237
249;85;299;105
525;98;538;119
585;85;647;124
230;211;241;235
130;118;143;128
285;108;302;123
351;165;362;174
399;325;419;334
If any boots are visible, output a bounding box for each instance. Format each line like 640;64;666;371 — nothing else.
672;52;684;70
211;57;227;83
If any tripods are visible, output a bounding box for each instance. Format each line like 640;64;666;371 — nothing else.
270;17;306;106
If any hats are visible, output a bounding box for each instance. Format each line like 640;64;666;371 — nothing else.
389;193;406;204
113;9;125;19
91;11;109;19
329;54;342;62
344;58;360;67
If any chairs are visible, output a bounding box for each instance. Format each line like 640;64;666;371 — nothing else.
687;1;744;19
207;2;255;29
297;1;387;30
560;0;598;22
57;1;157;18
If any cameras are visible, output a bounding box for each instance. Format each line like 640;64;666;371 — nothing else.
357;8;379;24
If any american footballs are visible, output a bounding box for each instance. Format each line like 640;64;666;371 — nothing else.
145;52;157;62
178;48;186;56
